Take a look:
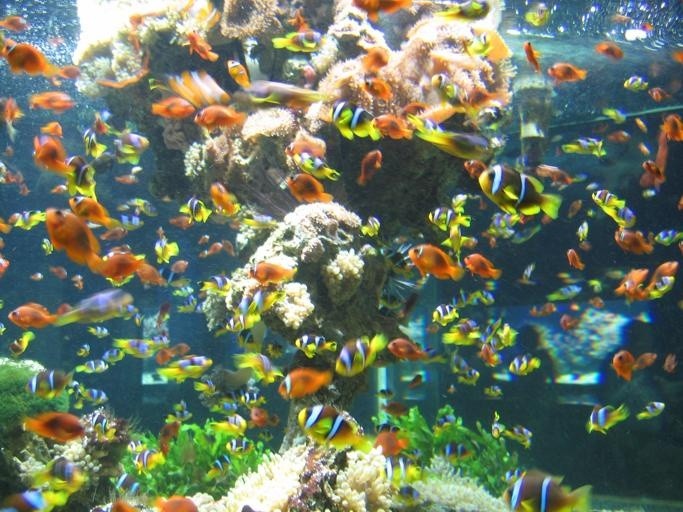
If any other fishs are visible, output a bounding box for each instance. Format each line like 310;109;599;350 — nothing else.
0;0;683;512
